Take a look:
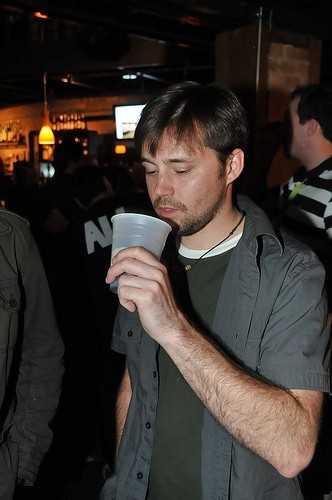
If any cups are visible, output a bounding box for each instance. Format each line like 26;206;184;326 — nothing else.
109;213;170;292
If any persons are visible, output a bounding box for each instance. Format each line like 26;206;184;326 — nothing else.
98;79;332;500
0;81;332;500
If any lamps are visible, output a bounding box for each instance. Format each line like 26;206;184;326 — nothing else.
38;72;56;145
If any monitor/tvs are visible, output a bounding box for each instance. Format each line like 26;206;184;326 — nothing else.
112;103;146;140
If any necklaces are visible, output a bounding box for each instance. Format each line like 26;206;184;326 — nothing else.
185;210;247;272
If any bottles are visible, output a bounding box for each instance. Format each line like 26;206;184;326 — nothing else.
0;120;25;143
51;113;86;130
0;147;26;172
